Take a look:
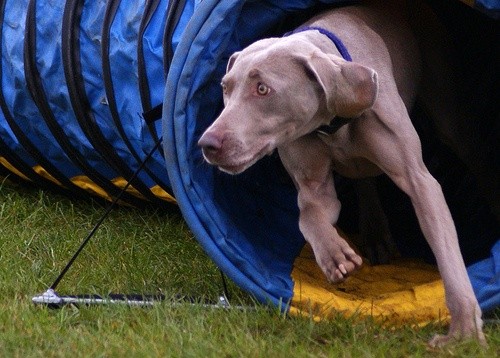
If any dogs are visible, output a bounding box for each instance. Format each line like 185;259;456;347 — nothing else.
198;0;489;353
